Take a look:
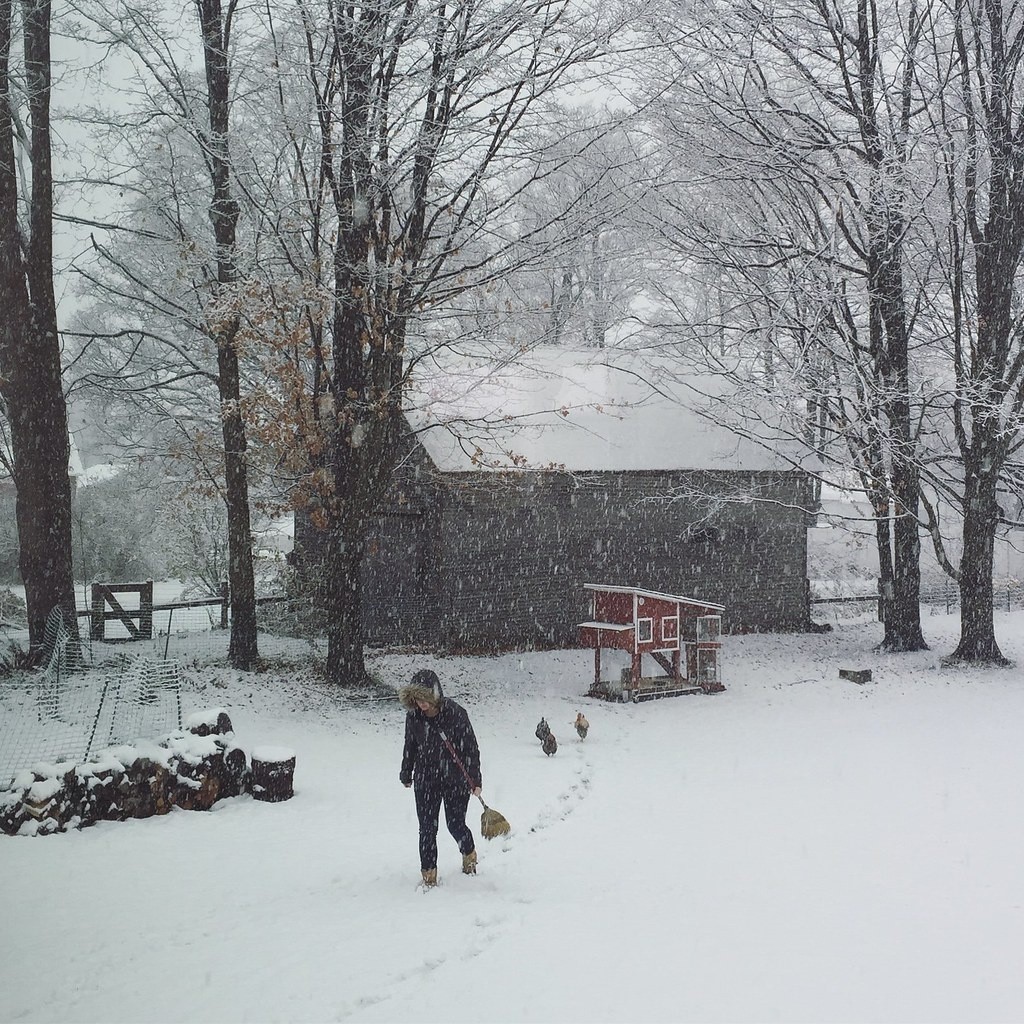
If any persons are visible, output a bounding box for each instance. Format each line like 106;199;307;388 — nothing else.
399;669;482;885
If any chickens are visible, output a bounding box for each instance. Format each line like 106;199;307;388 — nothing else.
575;713;589;740
542;732;558;756
535;717;551;744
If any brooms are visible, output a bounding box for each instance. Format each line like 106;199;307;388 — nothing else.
435;727;511;844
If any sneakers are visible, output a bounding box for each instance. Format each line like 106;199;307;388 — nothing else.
420;867;438;890
460;849;478;876
